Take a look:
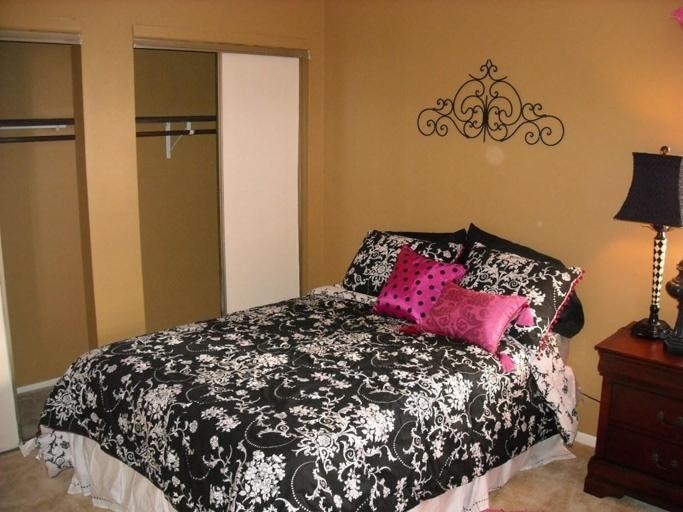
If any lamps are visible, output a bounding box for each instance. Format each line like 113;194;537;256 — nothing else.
612;144;683;341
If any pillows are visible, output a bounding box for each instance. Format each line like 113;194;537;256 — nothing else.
372;245;468;324
343;229;465;297
400;283;533;373
455;222;585;349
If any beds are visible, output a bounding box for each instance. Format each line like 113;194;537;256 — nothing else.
18;284;578;512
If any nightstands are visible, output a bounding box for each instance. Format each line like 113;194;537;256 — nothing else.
583;320;683;512
